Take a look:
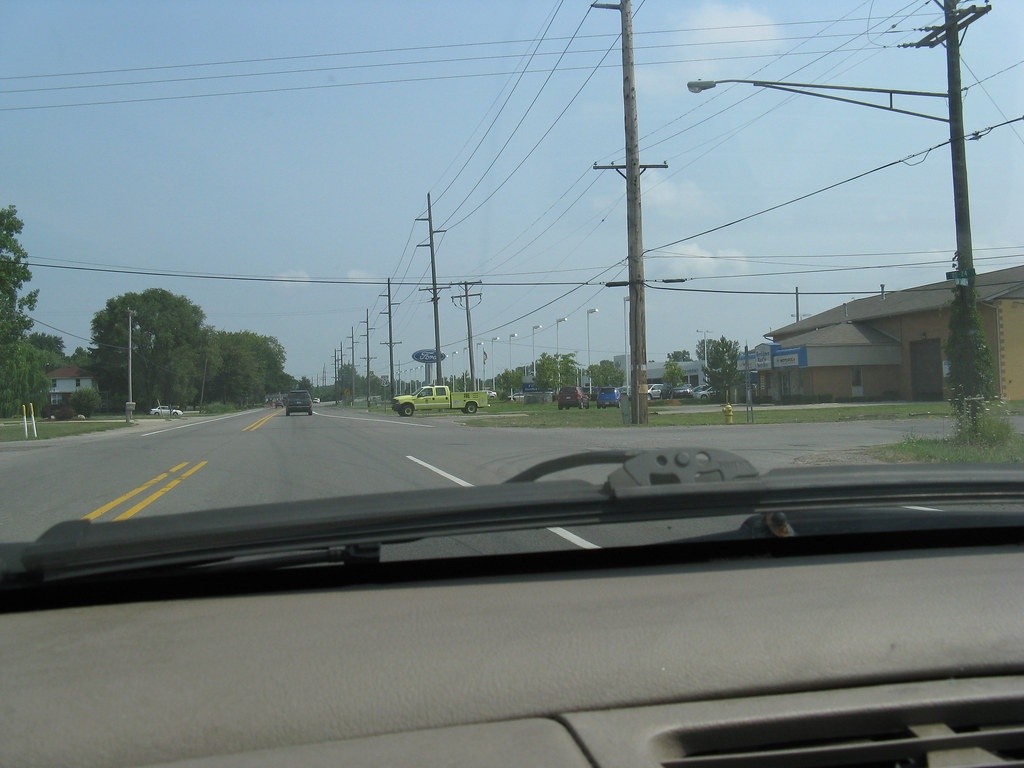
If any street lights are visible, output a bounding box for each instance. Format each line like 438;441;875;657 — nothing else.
509;333;518;373
687;78;985;440
410;368;414;395
556;318;567;359
491;336;500;391
403;370;408;395
585;307;598;369
452;351;459;392
414;367;419;391
532;325;543;377
623;296;631;397
475;342;484;387
419;365;424;388
462;347;469;392
697;330;707;384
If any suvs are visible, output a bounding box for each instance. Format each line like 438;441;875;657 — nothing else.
284;389;314;416
597;387;622;408
558;386;590;410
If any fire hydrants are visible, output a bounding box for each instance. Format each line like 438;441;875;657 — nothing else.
722;401;734;425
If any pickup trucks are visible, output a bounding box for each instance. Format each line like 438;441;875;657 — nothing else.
392;384;491;417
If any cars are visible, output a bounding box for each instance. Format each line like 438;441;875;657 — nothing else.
483;390;497;400
646;383;717;401
149;405;184;418
508;392;525;401
274;398;284;408
313;397;321;403
